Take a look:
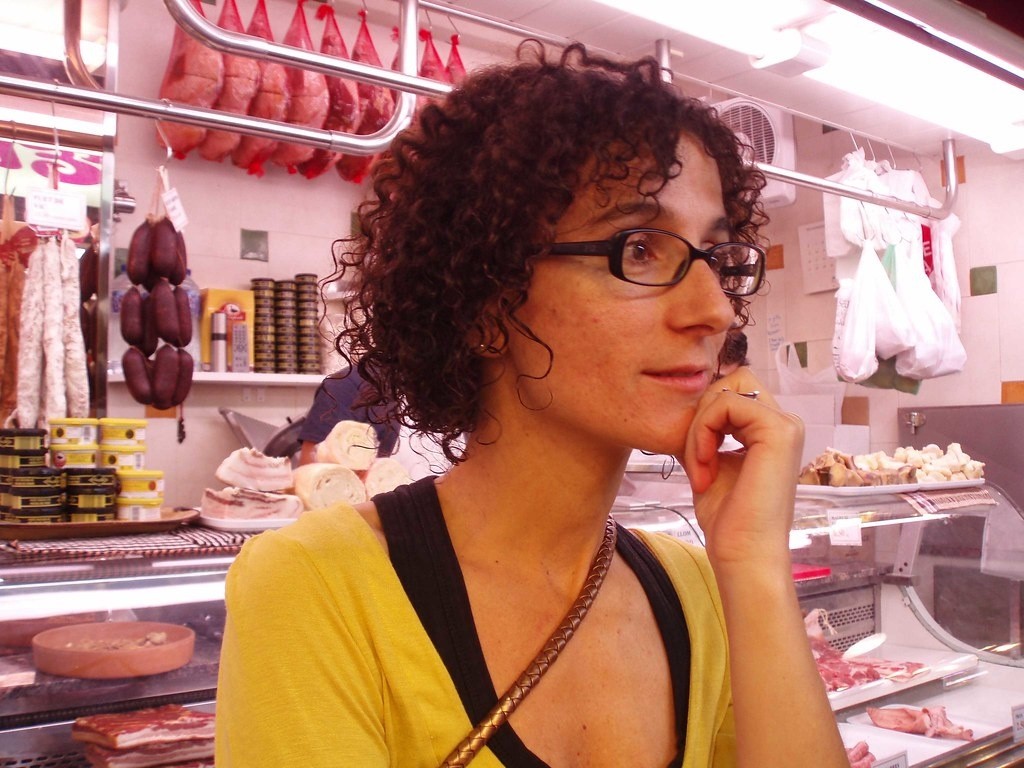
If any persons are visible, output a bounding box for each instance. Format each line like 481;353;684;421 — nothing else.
214;40;851;768
296;356;401;483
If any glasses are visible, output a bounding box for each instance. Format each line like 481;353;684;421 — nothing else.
524;226;765;299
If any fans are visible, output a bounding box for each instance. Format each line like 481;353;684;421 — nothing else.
708;95;798;208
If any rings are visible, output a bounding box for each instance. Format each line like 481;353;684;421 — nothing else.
723;388;760;401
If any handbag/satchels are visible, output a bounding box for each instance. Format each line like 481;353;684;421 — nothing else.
832;229;968;386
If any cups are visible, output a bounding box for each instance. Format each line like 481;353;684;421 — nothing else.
211;312;226;374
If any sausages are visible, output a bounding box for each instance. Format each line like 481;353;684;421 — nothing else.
0;228;92;430
79;241;100;391
120;217;194;409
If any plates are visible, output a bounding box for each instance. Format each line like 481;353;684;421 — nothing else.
32;621;196;680
192;506;298;533
